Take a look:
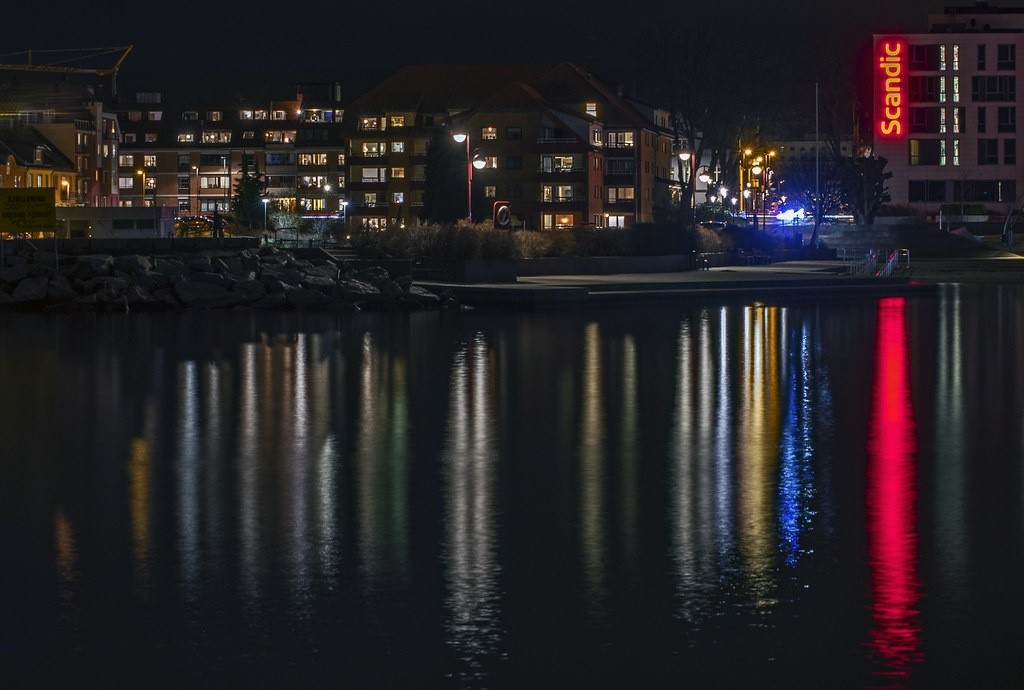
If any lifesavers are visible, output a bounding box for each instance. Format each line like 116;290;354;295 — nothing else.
496;205;512;225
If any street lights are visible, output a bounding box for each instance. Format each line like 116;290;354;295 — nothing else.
738;147;754;213
222;156;228;215
138;170;146;208
190;166;200;218
62;181;70;204
262;199;270;232
675;148;695;221
761;150;775;230
341;201;348;227
449;124;473;222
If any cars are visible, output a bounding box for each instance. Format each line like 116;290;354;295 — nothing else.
174;216;229;232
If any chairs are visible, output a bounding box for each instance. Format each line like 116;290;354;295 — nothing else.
737;248;766;265
